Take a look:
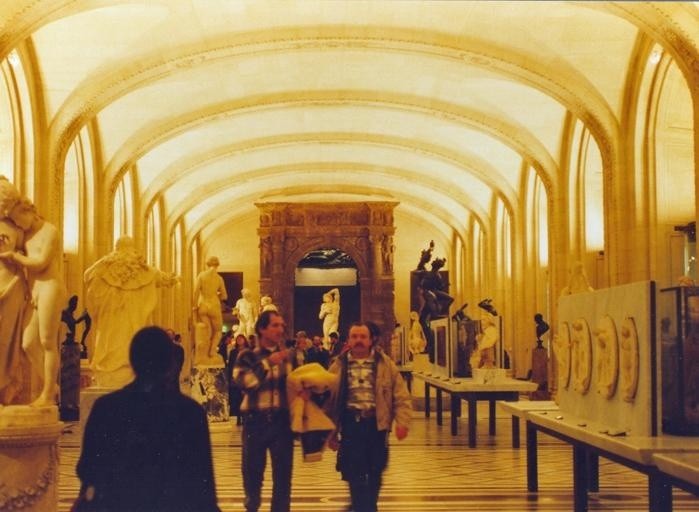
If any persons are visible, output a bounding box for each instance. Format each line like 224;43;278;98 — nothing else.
260;296;279;313
318;288;340;350
416;249;454;315
534;313;550;338
410;258;446;354
84;236;182;389
192;256;228;357
226;311;414;512
0;179;46;406
75;326;223;512
13;198;66;408
232;289;259;337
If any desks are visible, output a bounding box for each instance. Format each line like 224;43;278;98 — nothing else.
501;401;558;448
526;410;699;512
653;452;699;512
413;371;538;448
